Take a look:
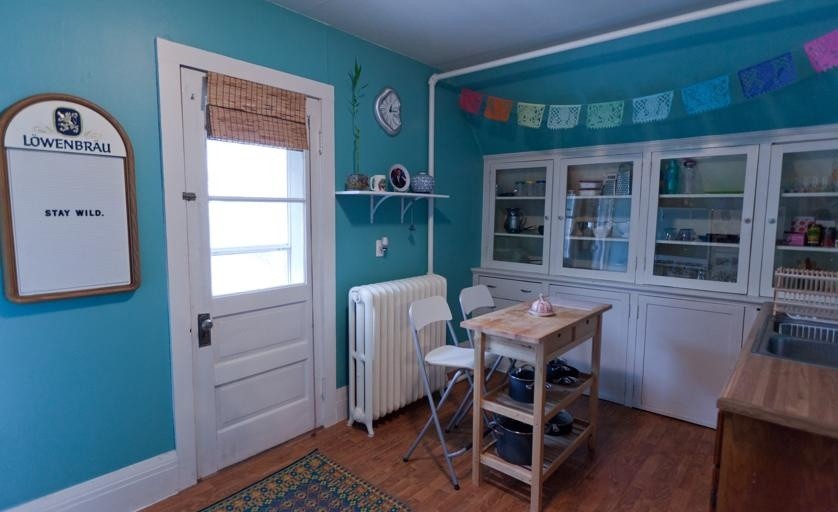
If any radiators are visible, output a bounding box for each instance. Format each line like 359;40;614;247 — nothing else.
345;274;447;439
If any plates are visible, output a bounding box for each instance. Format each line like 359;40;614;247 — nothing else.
388;162;411;192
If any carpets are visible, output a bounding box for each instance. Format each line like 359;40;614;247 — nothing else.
193;448;414;512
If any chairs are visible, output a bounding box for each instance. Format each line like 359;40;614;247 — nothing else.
402;284;520;490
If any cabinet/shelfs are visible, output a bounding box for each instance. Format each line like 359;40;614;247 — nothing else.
459;295;612;512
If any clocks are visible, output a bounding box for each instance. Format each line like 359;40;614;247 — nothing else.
373;87;402;136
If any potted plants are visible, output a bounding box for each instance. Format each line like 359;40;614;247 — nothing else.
344;54;368;190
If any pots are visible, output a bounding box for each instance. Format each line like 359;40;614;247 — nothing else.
543;408;575;437
507;363;551;403
486;411;533;467
543;363;589;388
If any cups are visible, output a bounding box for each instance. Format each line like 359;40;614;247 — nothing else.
661;227;697;241
368;174;387;192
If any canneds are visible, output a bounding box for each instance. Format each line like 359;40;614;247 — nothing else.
807;223;822;246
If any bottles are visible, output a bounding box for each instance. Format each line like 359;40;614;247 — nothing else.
682;160;704;194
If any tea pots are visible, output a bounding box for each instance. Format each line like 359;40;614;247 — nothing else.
502;207;528;234
